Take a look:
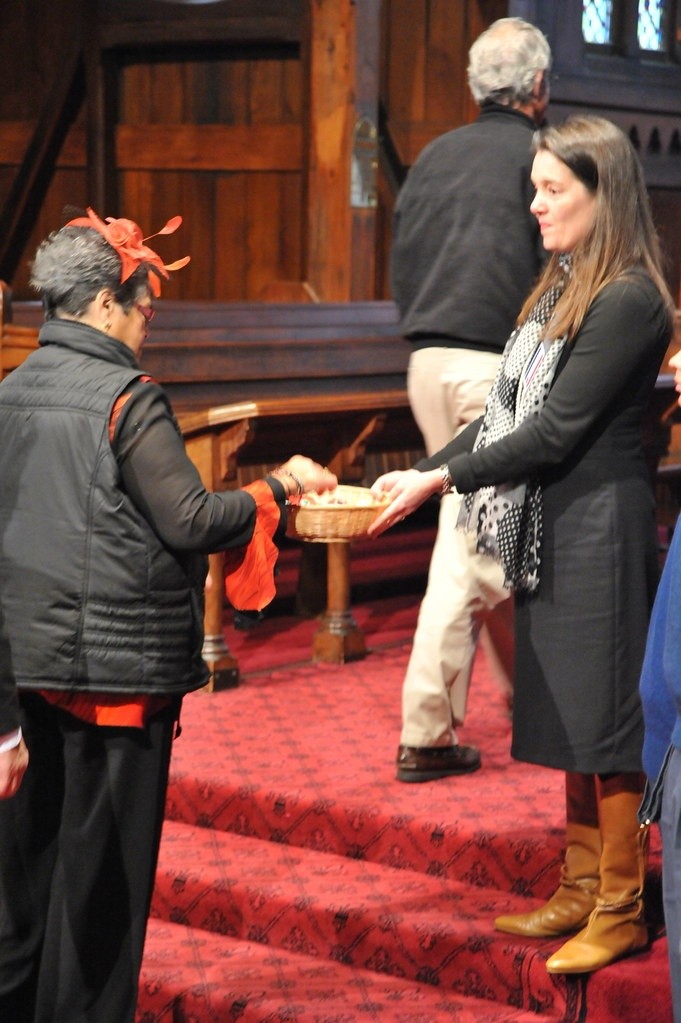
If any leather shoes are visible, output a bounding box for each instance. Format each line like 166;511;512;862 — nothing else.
396;744;481;782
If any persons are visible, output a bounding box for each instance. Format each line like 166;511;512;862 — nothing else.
396;19;559;784
368;115;678;975
0;209;336;1022
639;308;681;1022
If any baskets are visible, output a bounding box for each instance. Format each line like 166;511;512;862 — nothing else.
284;483;392;544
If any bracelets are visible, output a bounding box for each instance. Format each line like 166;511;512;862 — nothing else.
0;726;22;752
268;466;303;505
440;464;450;496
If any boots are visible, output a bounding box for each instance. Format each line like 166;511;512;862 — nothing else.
495;771;603;937
545;771;650;974
